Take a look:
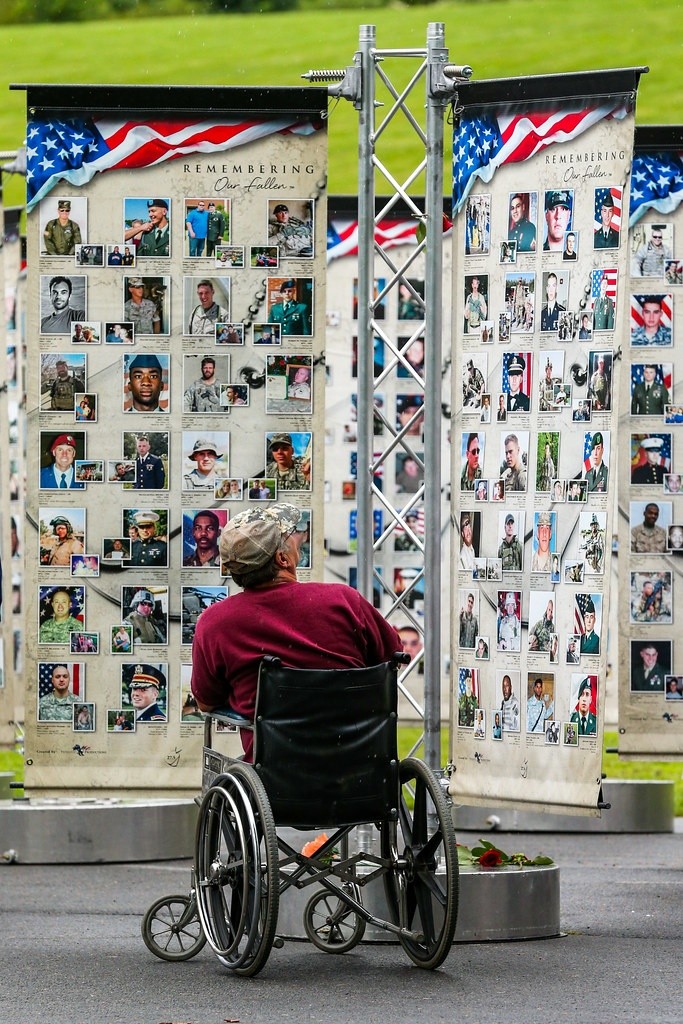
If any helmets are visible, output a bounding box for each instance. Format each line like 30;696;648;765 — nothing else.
129;590;155;611
568;638;576;644
505;593;516;609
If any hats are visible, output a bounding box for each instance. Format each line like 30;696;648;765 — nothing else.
545;190;614;211
127;276;295;293
49;433;292;461
55;524;66;530
578;677;592;699
58;200;288;214
464;669;472;679
50;511;160;535
129;355;163;370
505;512;599;538
219;503;302;575
466;355;658;380
129;664;166;692
590;432;664;451
584;594;595;613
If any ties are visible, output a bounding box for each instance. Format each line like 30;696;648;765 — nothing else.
645;669;649;679
141;458;144;464
593;469;596;484
284;304;289;313
581;716;586;735
60;473;67;488
155;230;162;245
646;385;650;393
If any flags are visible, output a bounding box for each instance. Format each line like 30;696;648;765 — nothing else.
452;100;632;215
629;153;683;228
327;221;426;264
26;118;317;212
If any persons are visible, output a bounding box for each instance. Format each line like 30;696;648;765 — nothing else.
351;188;683;743
38;198;315;732
190;502;406;822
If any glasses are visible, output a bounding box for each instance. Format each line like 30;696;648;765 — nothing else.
139;601;154;607
468;448;480;455
198;204;205;207
130;285;144;289
59;208;70;212
283;525;298;543
652;236;662;239
469;600;473;604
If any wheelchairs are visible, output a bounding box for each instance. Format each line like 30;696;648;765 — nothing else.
141;653;460;982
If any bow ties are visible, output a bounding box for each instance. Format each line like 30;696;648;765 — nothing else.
509;394;518;400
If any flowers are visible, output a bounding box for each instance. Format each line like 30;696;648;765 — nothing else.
301;832;340;869
457;839;553;870
